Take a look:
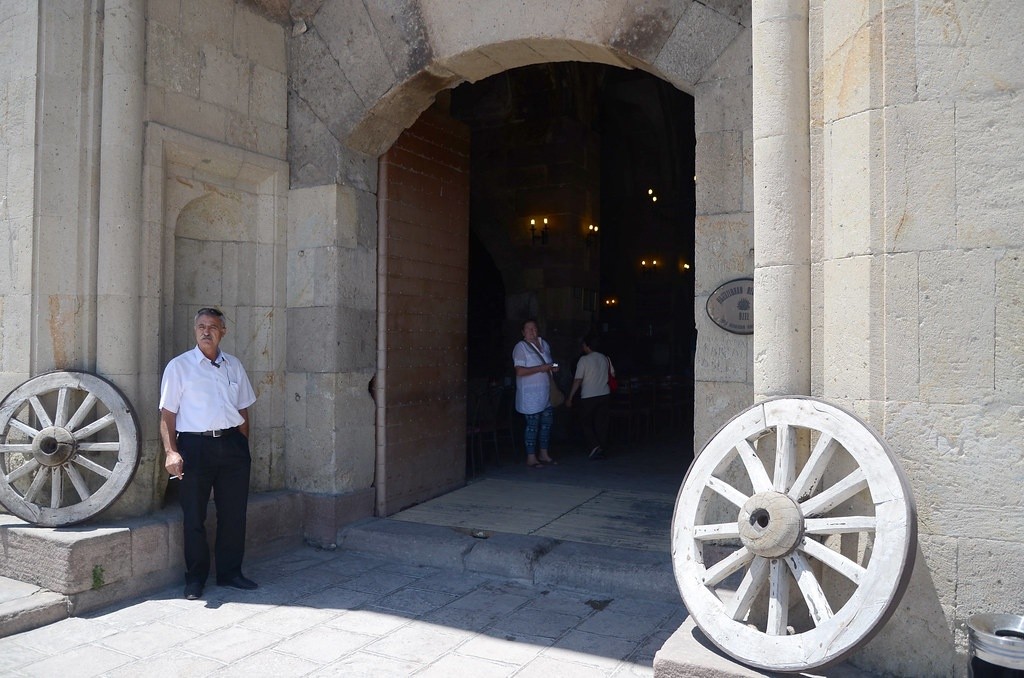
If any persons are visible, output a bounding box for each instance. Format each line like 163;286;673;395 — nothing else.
159;308;258;600
564;336;616;460
512;318;560;469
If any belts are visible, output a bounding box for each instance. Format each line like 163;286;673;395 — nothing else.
183;428;239;438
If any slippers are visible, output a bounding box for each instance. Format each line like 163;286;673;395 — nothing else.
539;458;559;466
526;463;544;469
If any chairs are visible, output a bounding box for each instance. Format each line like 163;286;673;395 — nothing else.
467;385;525;480
608;382;648;446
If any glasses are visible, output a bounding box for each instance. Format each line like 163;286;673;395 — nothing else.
197;307;224;317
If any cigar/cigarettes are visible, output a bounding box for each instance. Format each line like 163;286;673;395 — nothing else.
169;473;184;479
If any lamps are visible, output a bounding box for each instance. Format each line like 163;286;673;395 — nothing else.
529;216;551;246
584;223;600;247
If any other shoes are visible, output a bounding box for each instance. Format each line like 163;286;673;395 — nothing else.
184;581;202;600
587;443;601;459
216;573;258;590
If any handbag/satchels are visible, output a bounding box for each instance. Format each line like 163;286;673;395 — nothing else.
549;379;565;408
609;374;619;394
